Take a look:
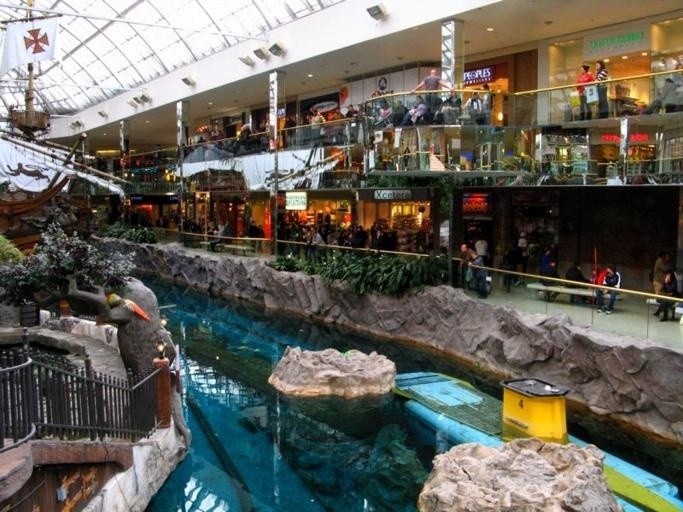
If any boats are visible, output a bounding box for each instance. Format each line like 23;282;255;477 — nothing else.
394;369;683;511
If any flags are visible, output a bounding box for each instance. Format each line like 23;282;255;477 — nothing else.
0;19;60;82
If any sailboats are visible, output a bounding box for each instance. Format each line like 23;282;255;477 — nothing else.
0;0;136;253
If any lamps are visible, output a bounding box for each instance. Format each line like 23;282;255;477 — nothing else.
127;92;152;108
268;42;288;58
253;46;271;61
98;110;109;118
238;54;255;67
366;3;389;20
181;76;196;87
68;119;85;131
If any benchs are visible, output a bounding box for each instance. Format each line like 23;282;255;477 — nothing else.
527;282;628;311
646;297;683;321
200;242;224;252
224;244;253;257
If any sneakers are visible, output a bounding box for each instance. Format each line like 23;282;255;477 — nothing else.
596;308;612;315
